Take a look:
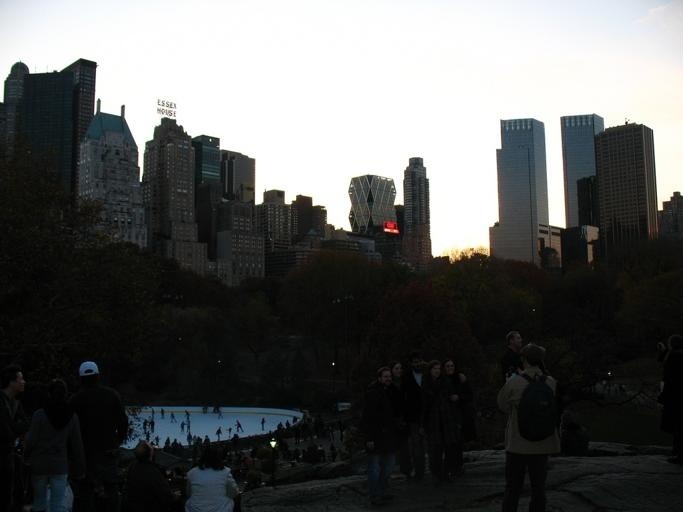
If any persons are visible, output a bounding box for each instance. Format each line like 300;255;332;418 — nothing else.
360;360;476;506
123;404;352;491
497;342;561;512
0;366;30;512
500;331;523;383
22;377;85;512
68;360;128;512
656;335;683;465
123;441;241;510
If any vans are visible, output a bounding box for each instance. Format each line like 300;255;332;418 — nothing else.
338;402;351;413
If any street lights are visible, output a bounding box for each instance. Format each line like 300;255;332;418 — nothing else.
270;437;278;472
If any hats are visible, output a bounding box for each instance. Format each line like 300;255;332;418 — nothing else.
78;361;98;377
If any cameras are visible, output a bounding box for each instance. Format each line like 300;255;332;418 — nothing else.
509;371;519;377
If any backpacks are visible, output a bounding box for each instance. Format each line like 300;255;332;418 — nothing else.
513;375;557;441
